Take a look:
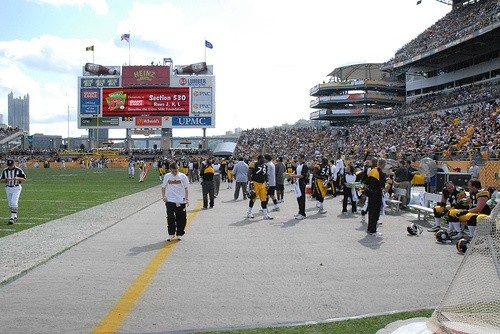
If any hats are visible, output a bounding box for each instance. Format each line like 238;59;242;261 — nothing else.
7;160;14;167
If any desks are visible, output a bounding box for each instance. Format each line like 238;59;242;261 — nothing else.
436;172;473;192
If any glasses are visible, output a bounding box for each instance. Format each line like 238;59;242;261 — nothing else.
298;158;301;159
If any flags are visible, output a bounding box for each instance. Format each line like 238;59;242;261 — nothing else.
85;46;94;51
205;40;212;49
121;34;129;42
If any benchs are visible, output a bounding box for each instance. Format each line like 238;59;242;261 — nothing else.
407;193;448;222
385;187;406;212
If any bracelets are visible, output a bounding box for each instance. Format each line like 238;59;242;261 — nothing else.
6;179;8;181
186;199;189;201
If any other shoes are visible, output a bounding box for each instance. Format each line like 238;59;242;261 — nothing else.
378;222;383;226
293;214;306;220
9;217;18;224
317;204;320;208
201;206;213;210
247;213;254;218
263;215;274;220
319;209;328;214
277;199;284;203
369;231;383;238
448;231;462;240
402;205;407;208
426;226;441;232
407;224;423;236
177;235;183;240
271;207;280;212
361;214;365;221
159;176;162;180
167;235;175;241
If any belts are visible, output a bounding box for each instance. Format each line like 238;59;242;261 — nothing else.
6;185;19;187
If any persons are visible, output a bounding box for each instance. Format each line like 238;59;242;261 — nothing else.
0;0;500;248
